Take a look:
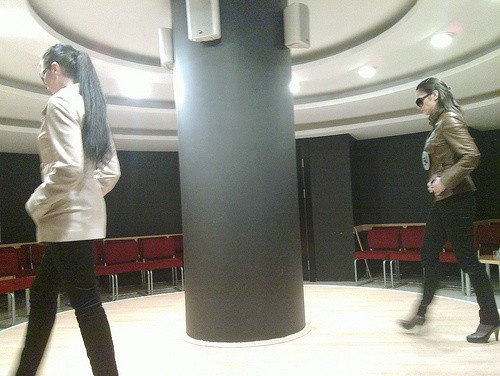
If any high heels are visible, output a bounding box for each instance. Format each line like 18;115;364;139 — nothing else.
467;323;498;343
398;314;426;333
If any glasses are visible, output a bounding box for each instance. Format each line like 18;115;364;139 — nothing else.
38;64;51;83
416;92;431;106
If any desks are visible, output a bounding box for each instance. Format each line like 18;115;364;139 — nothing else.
478;255;500;282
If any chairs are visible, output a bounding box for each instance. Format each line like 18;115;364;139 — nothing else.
353;218;500;298
0;233;185;326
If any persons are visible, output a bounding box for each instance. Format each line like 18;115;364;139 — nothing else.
16;45;120;376
400;77;500;343
